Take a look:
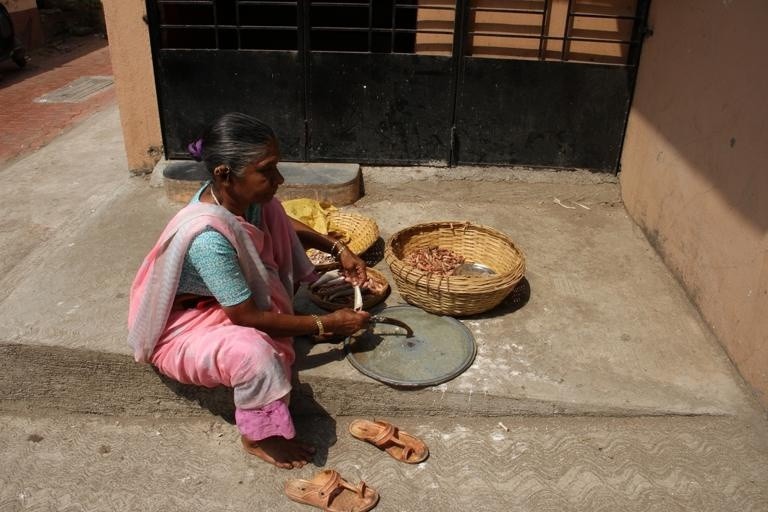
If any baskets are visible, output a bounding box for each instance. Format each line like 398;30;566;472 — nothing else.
302;208;389;311
384;220;526;318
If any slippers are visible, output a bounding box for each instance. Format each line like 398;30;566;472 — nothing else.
349;418;429;463
284;468;380;512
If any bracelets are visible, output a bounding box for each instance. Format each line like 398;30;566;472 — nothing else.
334;244;346;259
311;313;325;340
329;239;339;256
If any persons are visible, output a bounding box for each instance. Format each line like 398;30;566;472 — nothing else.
126;110;375;474
